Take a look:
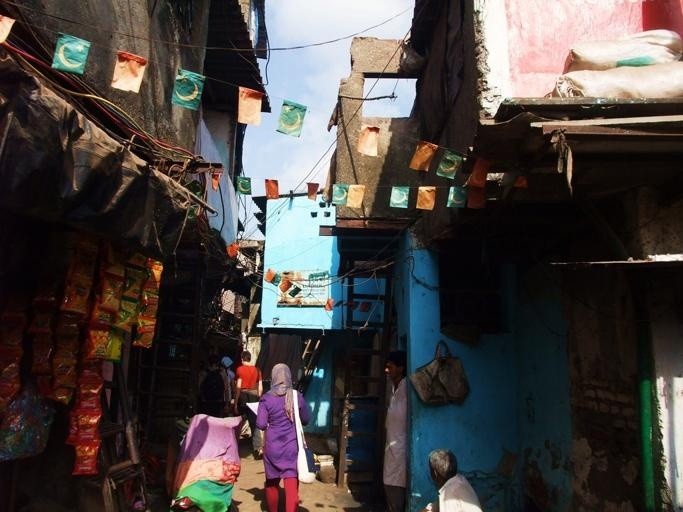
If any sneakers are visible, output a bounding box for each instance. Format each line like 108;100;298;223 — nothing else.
255;448;264;460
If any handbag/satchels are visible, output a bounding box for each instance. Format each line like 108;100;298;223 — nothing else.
297;448;320;483
410;341;468;406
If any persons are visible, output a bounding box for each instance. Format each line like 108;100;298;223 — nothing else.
255;364;310;512
198;351;264;434
382;350;407;512
419;449;483;512
